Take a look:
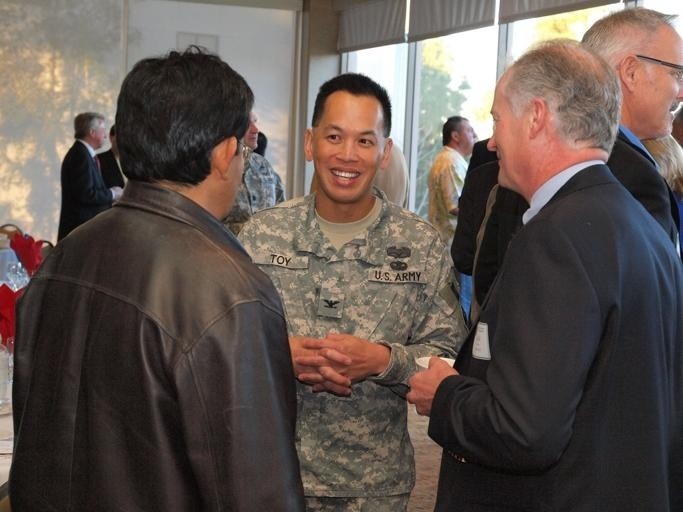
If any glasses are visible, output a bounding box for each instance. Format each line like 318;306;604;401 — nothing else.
214;134;248;158
636;54;683;82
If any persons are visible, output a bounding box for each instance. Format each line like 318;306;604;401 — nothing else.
427;115;479;250
309;143;408;208
406;38;682;512
449;137;498;277
7;49;302;512
96;123;129;190
236;71;471;512
473;6;682;328
57;111;123;243
221;110;287;238
